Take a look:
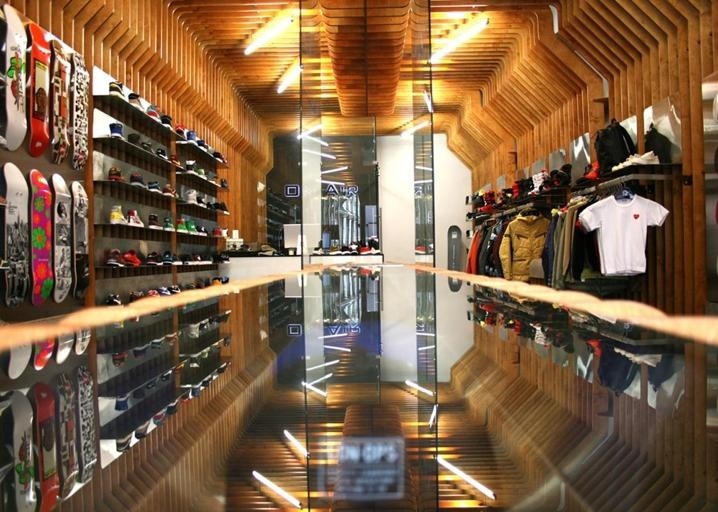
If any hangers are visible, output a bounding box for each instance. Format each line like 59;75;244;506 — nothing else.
469;176;635;238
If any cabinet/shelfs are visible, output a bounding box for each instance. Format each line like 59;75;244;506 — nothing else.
0;4;231;318
1;291;230;511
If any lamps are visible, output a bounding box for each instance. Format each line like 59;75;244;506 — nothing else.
249;330;497;511
243;15;486;145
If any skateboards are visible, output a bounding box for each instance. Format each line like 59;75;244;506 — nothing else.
448;225;462;270
448;277;462;292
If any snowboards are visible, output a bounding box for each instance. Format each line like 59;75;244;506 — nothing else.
1;3;89;171
1;160;90;310
9;364;97;512
8;327;91;379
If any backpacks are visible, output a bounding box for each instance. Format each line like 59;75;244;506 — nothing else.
648;345;674;392
594;119;638;178
645;128;671;163
596;339;639;397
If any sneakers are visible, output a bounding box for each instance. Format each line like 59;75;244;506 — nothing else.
415;245;427;255
324;267;381;281
328;240;381;255
105;81;231;305
112;299;231;454
311;247;325;256
611;151;661;173
614;347;663;368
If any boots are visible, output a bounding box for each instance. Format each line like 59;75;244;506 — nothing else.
570;160;601;193
575;327;614;358
467;163;571;226
473;301;573;353
